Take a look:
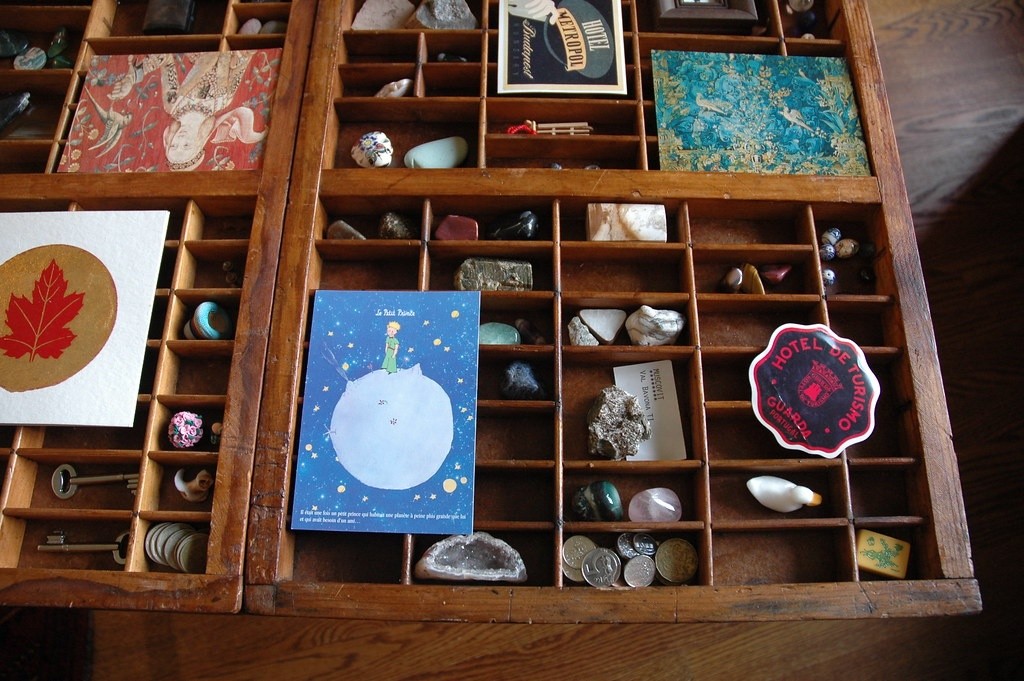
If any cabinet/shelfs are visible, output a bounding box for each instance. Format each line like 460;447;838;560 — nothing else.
0;0;981;622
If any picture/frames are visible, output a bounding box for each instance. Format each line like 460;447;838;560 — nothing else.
498;0;628;95
